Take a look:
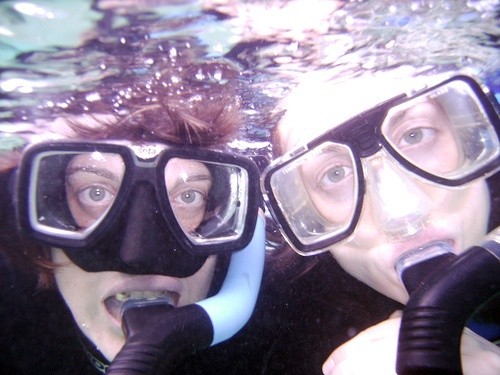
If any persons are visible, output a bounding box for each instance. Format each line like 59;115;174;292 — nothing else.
0;40;500;375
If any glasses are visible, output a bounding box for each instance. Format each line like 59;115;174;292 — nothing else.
7;139;262;258
258;74;500;256
